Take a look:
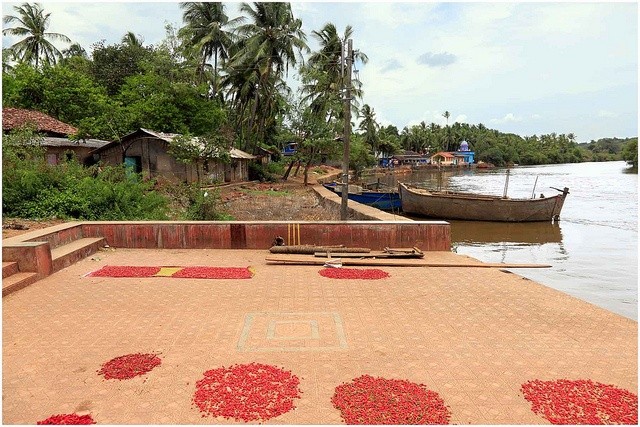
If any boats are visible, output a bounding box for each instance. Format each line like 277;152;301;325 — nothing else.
397;181;570;222
322;181;402;210
